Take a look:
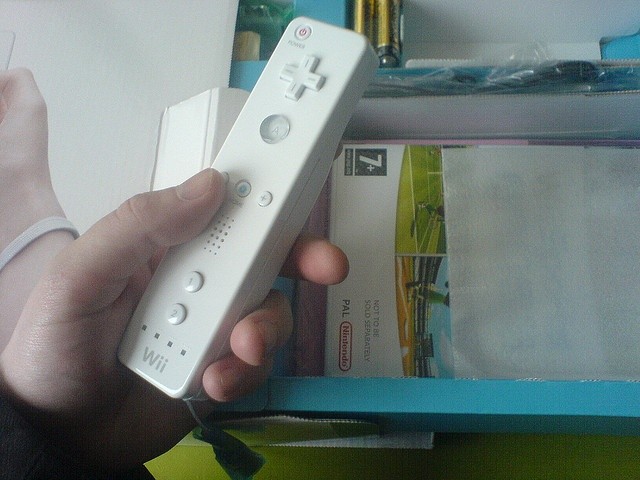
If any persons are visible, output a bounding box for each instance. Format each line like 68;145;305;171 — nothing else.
0;169;350;475
1;67;80;359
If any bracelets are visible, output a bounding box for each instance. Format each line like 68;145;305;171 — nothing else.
0;218;81;273
